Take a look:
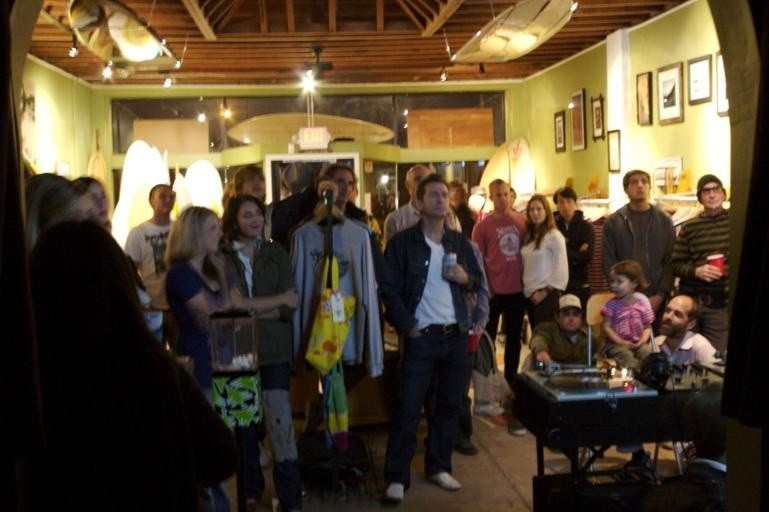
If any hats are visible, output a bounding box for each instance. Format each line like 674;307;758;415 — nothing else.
696;173;721;197
557;293;583;313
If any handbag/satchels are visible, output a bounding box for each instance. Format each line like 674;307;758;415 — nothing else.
472;329;516;405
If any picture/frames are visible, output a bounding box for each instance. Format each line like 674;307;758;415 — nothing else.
656;62;684;127
687;54;712;105
606;130;620;172
590;93;604;143
635;72;652;126
569;88;585;152
554;110;565;153
716;51;729;117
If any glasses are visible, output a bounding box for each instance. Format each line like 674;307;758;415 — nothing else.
703;185;721;192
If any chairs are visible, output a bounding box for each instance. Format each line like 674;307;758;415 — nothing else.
586;292;656;366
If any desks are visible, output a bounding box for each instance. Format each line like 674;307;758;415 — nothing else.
512;361;723;485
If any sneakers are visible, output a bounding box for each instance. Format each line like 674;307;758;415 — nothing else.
455;439;478;456
473;402;505;417
509;415;527;437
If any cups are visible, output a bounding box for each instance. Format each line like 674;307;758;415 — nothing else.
706;254;725;276
468;330;479;353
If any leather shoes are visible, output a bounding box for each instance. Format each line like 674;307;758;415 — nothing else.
426;471;462;490
384;481;405;503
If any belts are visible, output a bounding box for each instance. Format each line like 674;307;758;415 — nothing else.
421;323;459;334
697;292;726;308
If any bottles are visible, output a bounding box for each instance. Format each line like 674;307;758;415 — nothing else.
444;251;457;274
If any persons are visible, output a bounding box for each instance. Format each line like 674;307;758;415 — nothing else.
448;181;477;238
498;189;528;346
597;262;655;372
520;194;569;336
221;194;304;512
165;207;239;511
454;239;490;457
237;168;267;204
272;161;317;253
601;170;677;311
125;185;180;350
553;187;596;297
22;172;94;249
616;295;725;472
321;165;369;227
509;295;596;438
669;174;730;355
381;175;484;501
384;165;462;249
472;180;527;386
32;222;239;511
74;176;110;231
